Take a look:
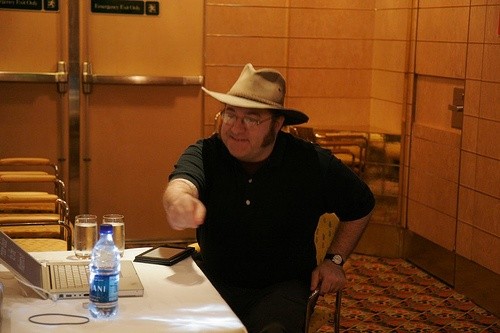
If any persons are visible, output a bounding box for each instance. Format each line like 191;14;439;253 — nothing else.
162;63;375;333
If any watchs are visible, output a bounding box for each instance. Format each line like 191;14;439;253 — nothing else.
325;254;344;266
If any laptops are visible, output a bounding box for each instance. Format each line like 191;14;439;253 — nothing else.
0;231;142;301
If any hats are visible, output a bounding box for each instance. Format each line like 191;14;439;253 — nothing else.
201;62;311;127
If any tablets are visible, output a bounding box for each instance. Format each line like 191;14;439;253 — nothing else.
133;245;196;266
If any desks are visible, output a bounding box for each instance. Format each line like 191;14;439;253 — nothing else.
0;246;249;333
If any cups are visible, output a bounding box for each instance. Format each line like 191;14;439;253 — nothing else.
74;213;98;259
102;214;125;257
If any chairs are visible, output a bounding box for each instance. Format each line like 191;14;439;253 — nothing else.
0;158;74;252
291;126;402;197
188;212;342;333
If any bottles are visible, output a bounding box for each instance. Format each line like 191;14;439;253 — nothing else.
88;224;121;319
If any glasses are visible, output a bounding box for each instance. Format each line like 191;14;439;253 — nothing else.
217;108;276;128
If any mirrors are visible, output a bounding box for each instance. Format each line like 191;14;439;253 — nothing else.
201;0;413;227
